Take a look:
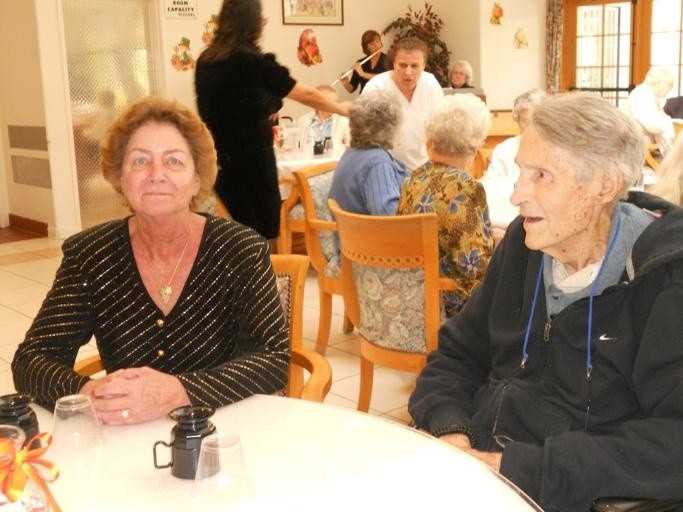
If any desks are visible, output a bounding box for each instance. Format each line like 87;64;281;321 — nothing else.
0;390;546;512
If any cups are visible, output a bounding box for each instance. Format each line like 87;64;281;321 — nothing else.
48;394;104;467
0;389;40;457
300;137;332;158
193;434;249;497
152;405;221;481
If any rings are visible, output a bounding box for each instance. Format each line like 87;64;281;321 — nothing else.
122;409;130;421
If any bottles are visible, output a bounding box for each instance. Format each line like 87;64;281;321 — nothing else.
0;420;48;512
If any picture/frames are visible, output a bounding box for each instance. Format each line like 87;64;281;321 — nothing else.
282;0;345;26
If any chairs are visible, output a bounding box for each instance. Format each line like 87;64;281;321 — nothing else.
271;168;306;255
327;197;459;412
292;160;352;354
70;251;331;403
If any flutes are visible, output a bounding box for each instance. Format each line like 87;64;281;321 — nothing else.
329;47;383;88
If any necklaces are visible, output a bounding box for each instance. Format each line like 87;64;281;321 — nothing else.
135;215;195;302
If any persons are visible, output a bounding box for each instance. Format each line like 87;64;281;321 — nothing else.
447;60;475;88
623;67;675;142
340;30;387;96
282;83;352;153
404;92;682;510
645;131;681;208
358;37;445;169
9;97;290;424
477;93;547;227
327;90;413;267
394;94;506;318
194;1;355;251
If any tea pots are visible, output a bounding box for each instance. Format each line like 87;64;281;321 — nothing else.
278;116;315;152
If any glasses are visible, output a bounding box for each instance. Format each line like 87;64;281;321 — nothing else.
493;364;593;449
